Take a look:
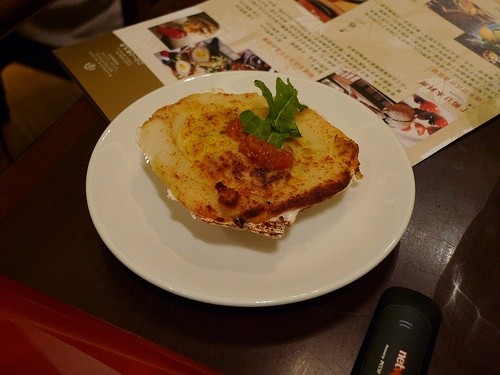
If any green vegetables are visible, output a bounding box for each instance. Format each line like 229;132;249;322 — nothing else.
239;76;309;149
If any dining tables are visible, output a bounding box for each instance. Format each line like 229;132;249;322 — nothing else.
0;0;499;374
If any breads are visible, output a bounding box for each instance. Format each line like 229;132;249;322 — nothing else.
136;91;360;225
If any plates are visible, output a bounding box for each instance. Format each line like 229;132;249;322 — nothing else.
84;69;419;308
